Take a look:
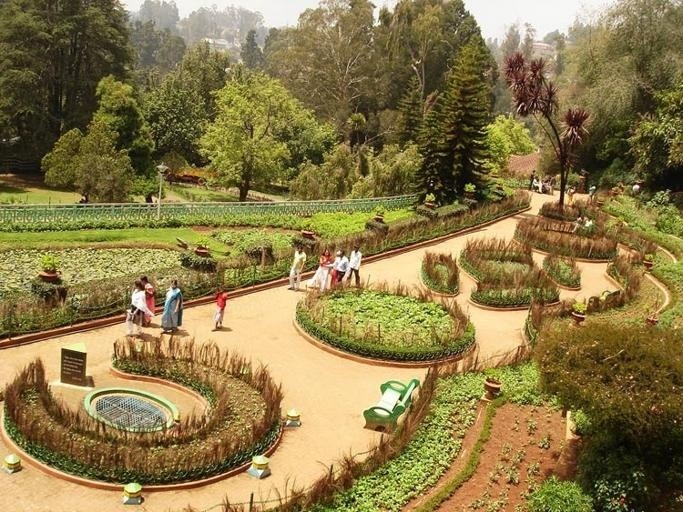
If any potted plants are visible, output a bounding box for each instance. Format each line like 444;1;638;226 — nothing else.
464;183;476;199
373;205;385;222
484;368;501;400
572;303;587;324
194;238;210;257
301;219;316;239
643;254;653;269
424;193;435;209
38;252;63;282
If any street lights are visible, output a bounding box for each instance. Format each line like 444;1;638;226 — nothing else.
154;162;171;218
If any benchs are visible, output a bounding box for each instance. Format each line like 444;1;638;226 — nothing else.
363;379;419;434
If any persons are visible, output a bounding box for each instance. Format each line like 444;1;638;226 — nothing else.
567;184;576;203
160;279;183;333
588;184;597;200
213;284;227;331
530;168;551;194
140;276;155;324
287;245;306;291
310;244;362;293
129;279;146;325
